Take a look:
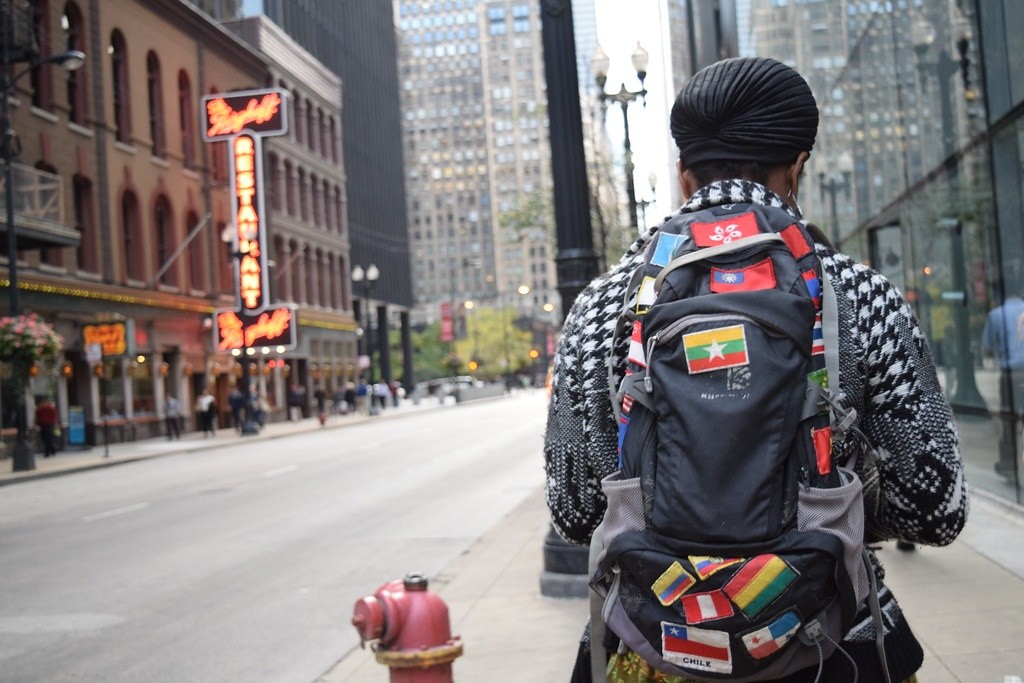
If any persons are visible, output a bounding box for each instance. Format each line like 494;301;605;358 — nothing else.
34;397;59;458
544;57;971;683
164;392;184;441
982;270;1024;489
288;376;402;427
228;384;245;431
193;384;216;439
245;383;265;428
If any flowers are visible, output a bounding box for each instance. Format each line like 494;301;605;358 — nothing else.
0;311;65;364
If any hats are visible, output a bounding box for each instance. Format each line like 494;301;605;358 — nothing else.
668;57;821;168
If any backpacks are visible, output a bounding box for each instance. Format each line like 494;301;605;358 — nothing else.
586;202;892;683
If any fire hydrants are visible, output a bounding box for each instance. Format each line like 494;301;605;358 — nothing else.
350;574;464;683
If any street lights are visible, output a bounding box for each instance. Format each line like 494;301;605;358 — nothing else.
589;37;648;247
1;49;89;473
352;261;381;414
906;0;990;416
